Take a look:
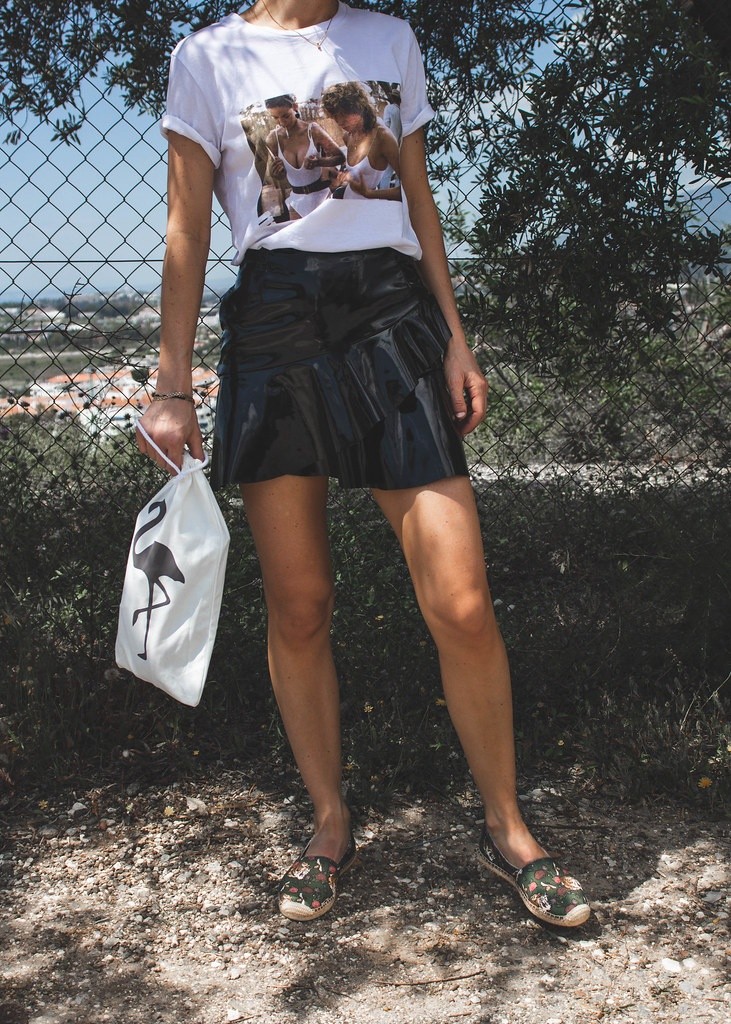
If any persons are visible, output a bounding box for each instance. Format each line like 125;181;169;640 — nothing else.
240;80;401;221
134;0;593;929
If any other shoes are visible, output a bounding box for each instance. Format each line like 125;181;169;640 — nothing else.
479;823;591;925
278;829;357;922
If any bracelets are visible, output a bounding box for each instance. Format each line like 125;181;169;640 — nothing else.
153;391;196;406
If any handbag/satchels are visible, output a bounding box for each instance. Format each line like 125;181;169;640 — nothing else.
115;419;231;707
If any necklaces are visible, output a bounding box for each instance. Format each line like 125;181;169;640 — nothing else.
261;0;340;52
